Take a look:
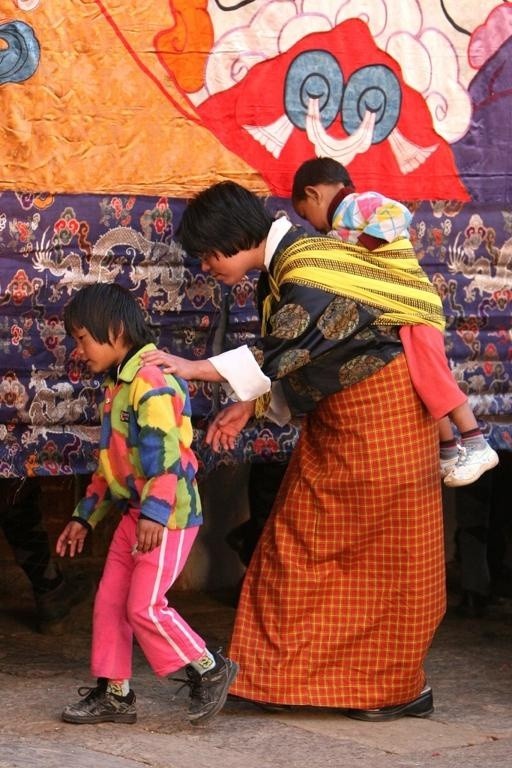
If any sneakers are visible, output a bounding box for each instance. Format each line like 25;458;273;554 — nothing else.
185;646;240;729
62;680;140;725
436;437;502;488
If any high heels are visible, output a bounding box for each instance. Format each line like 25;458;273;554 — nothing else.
342;682;433;721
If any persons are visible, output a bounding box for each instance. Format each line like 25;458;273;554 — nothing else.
137;177;448;720
53;283;241;724
288;154;502;489
